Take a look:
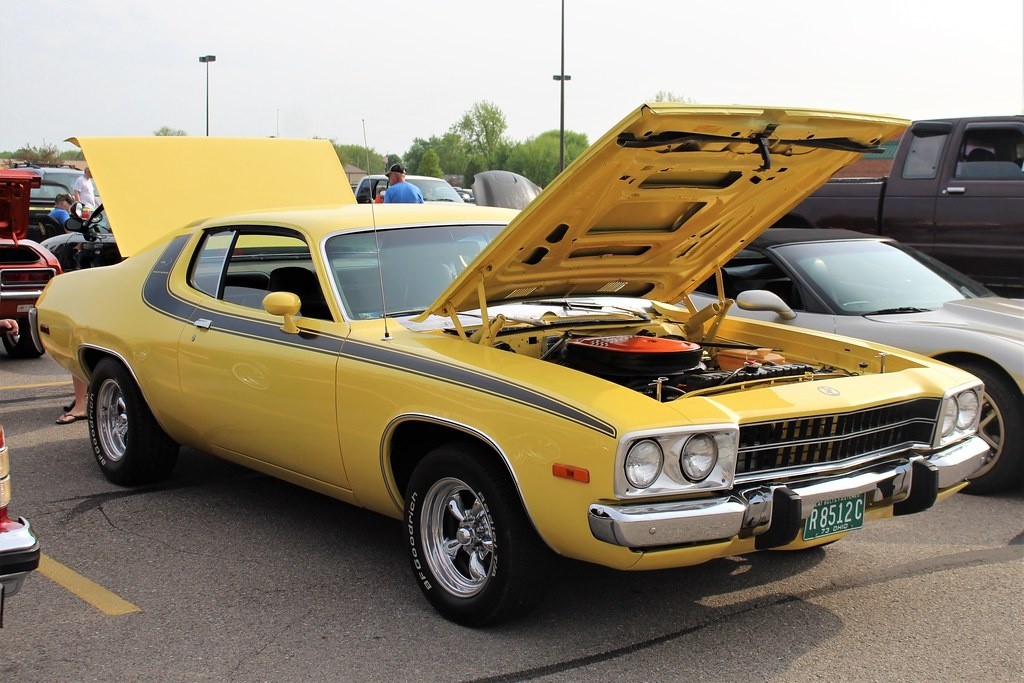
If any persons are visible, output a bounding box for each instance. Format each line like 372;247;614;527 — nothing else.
374;164;425;203
47;165;99;236
0;318;20;335
56;374;88;423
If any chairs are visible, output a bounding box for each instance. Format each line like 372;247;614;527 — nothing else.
377;237;458;311
698;269;737;299
961;148;996;178
259;266;333;321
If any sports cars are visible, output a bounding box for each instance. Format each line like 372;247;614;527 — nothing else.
32;99;992;631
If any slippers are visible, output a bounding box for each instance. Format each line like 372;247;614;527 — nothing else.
55;411;88;424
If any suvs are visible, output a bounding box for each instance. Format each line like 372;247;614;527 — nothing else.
10;162;109;228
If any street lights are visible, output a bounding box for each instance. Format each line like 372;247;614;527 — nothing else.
198;54;217;136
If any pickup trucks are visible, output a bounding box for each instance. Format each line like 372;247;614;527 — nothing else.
771;114;1024;299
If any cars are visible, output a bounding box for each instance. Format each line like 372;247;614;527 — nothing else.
0;166;65;359
28;180;76;243
37;200;128;274
355;169;544;251
673;228;1024;498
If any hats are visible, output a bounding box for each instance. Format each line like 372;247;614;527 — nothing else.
385;163;407;177
56;193;73;204
